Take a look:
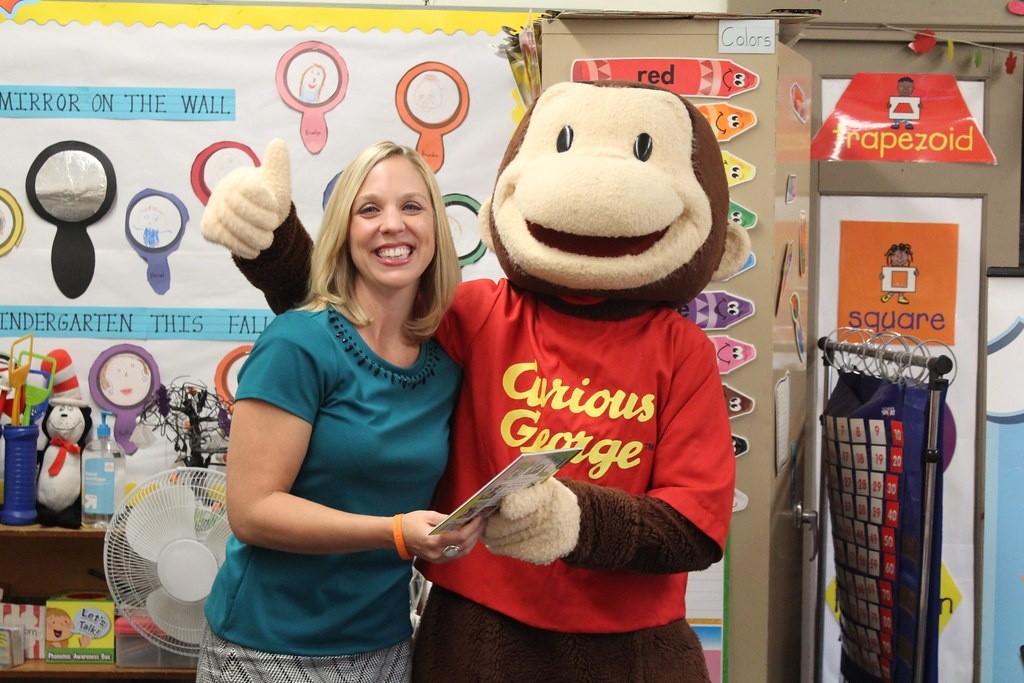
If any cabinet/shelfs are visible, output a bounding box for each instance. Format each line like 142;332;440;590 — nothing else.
0;519;199;683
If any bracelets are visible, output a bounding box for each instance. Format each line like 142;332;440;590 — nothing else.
392;512;414;561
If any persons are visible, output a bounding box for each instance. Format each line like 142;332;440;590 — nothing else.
195;138;489;683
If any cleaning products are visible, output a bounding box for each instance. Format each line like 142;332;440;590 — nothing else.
80;411;127;530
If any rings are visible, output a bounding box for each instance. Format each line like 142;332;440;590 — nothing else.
440;543;460;561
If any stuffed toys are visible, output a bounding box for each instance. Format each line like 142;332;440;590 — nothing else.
35;348;93;530
195;77;754;683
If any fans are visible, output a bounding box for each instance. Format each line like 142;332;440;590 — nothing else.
102;467;233;658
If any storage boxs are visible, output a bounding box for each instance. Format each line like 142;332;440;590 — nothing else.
0;591;198;670
